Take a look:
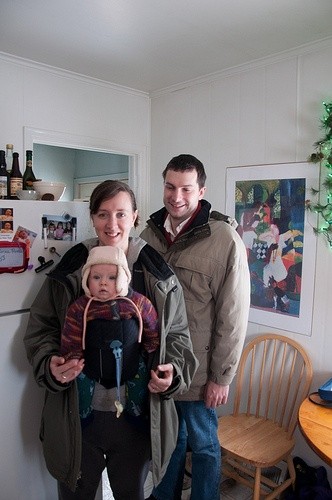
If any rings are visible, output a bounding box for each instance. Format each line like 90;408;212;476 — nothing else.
62;375;66;378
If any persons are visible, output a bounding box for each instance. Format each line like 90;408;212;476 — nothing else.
0;209;13;221
61;246;160;421
47;221;72;240
18;230;36;248
0;222;13;233
25;179;199;500
139;153;250;500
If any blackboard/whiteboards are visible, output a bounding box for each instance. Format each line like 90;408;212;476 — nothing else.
0;241;29;274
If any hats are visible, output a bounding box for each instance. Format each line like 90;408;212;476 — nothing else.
81;246;143;350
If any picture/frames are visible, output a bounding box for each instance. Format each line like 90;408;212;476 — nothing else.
225;160;320;337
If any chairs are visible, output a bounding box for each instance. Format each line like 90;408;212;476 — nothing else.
214;333;313;500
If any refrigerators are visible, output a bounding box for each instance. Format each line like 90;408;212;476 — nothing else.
0;199;103;500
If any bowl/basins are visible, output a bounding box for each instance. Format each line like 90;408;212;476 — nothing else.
15;189;40;201
32;181;67;201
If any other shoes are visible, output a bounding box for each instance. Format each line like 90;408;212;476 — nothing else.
80;412;94;429
123;409;150;433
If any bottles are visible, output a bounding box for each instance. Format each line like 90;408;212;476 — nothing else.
23;149;37;190
8;152;23;199
0;149;10;199
5;143;14;176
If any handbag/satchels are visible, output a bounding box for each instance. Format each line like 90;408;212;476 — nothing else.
280;457;332;500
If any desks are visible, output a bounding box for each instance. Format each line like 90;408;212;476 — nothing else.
297;393;332;466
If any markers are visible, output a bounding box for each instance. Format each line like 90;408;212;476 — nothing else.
73;226;75;247
44;224;48;249
